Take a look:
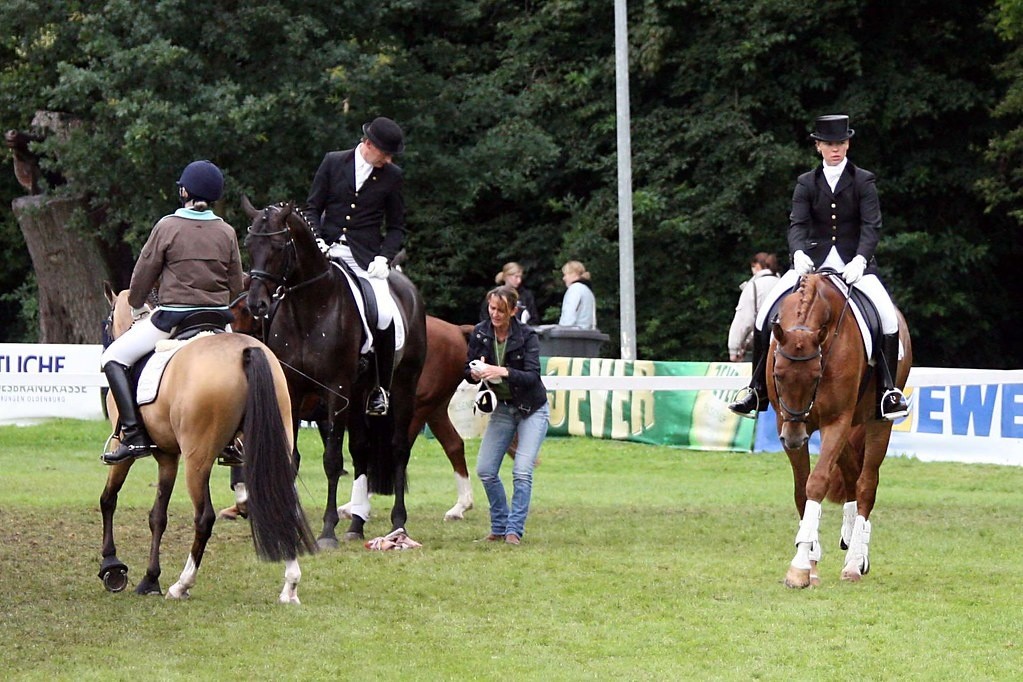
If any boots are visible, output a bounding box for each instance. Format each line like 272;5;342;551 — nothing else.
367;317;395;413
728;323;770;413
875;325;908;420
99;359;151;462
216;443;245;462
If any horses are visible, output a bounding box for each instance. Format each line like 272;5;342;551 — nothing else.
98;192;541;607
765;273;912;591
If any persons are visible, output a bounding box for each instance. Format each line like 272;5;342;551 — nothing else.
480;262;541;326
304;117;405;413
726;115;907;414
559;262;597;330
464;285;550;545
730;252;781;366
100;160;244;463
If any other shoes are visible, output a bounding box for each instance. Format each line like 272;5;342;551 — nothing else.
473;534;520;545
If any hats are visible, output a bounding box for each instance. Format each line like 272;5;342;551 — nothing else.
362;117;406;155
810;114;855;142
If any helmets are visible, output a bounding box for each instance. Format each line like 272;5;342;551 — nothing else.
175;159;224;202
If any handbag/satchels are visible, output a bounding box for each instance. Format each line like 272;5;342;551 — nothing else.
742;330;755;355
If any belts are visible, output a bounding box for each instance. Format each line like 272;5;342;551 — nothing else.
333;240;348;246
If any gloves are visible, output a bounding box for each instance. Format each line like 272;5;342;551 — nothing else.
841;254;867;284
367;255;390;280
793;250;814;276
316;238;329;253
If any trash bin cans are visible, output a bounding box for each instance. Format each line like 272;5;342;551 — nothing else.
532;325;610;358
